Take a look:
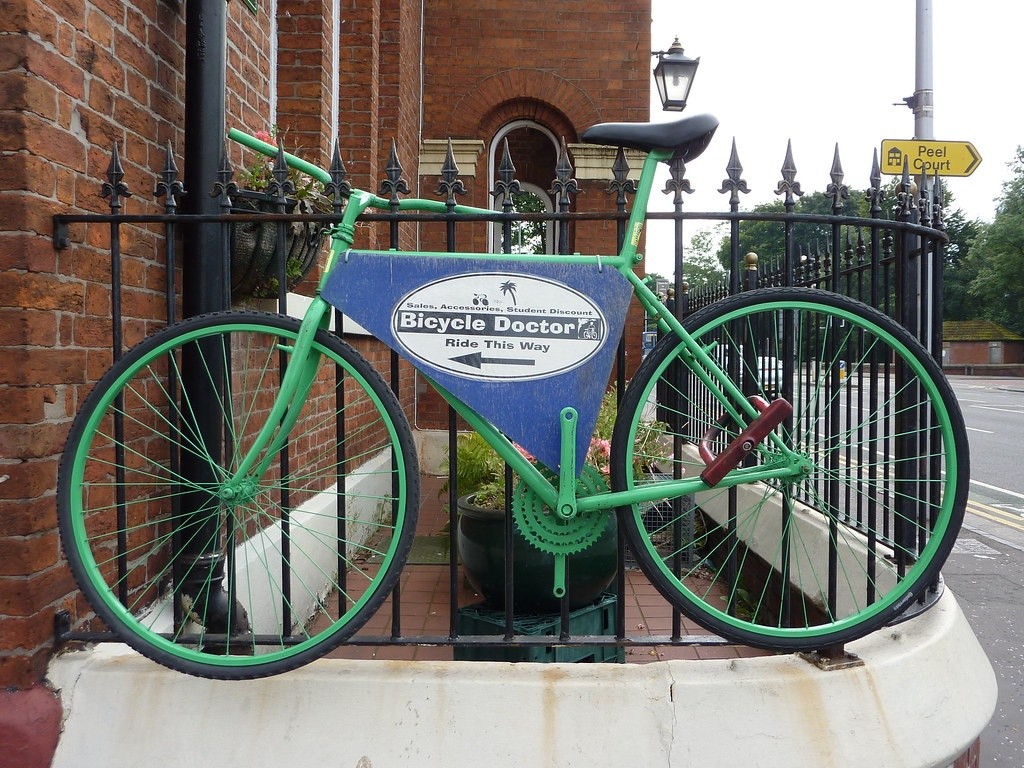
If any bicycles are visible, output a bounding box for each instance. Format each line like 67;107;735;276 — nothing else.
54;114;969;683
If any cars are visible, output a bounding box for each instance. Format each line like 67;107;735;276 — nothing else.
738;355;782;395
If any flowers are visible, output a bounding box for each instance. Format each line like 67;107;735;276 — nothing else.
236;123;335;239
473;437;611;506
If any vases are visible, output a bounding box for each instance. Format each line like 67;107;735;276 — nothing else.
457;492;618;614
230;189;333;298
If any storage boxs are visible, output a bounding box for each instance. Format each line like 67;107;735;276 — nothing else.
453;591;625;664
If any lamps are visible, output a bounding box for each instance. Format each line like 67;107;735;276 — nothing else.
651;34;701;111
